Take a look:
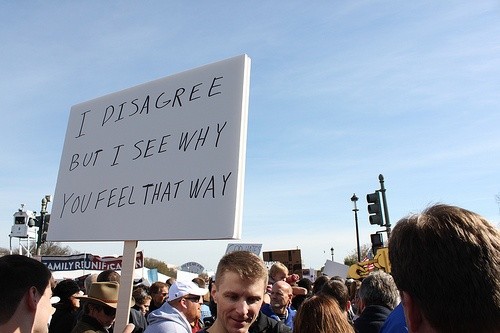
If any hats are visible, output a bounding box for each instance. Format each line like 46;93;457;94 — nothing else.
73;281;135;309
52;279;86;303
167;280;208;301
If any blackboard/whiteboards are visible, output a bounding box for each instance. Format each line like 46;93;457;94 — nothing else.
45;53;252;241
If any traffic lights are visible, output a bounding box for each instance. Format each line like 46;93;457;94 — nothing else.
370;233;384;257
42;214;51;242
366;191;384;227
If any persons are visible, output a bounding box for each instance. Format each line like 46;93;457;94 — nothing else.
0;252;410;333
148;281;170;310
387;201;500;333
259;281;297;328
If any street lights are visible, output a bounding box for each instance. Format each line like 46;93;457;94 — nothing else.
351;193;361;263
330;247;334;261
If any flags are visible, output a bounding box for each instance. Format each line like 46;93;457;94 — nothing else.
360;256;374;269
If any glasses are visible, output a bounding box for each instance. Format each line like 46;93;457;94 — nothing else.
179;296;201;303
161;292;168;296
100;308;116;316
